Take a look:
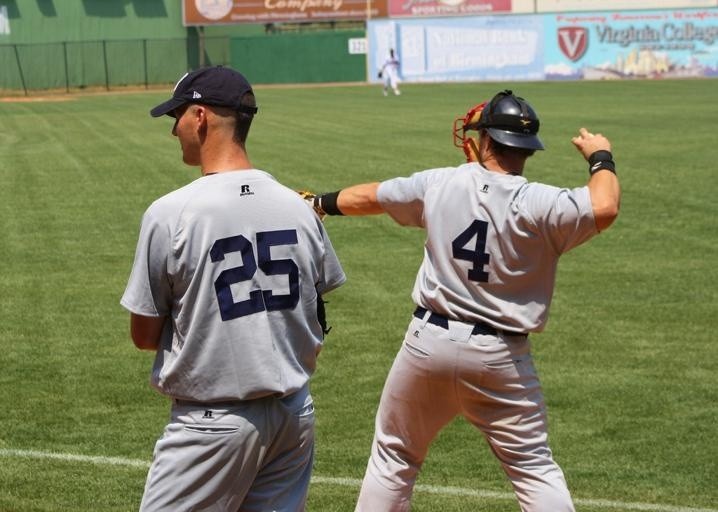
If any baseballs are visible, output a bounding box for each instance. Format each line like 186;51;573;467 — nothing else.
577;133;593;149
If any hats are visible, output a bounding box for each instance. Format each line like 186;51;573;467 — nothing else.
149;65;259;116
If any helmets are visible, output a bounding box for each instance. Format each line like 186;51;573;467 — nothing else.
452;91;545;166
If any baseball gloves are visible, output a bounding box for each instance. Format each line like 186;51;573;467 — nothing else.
379;71;383;77
295;189;327;221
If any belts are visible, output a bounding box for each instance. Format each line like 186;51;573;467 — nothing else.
411;303;527;339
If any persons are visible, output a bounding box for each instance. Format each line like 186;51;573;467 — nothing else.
301;86;621;512
117;62;347;512
378;47;403;97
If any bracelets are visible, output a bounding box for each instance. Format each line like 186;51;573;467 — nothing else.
588;149;617;175
320;189;343;217
